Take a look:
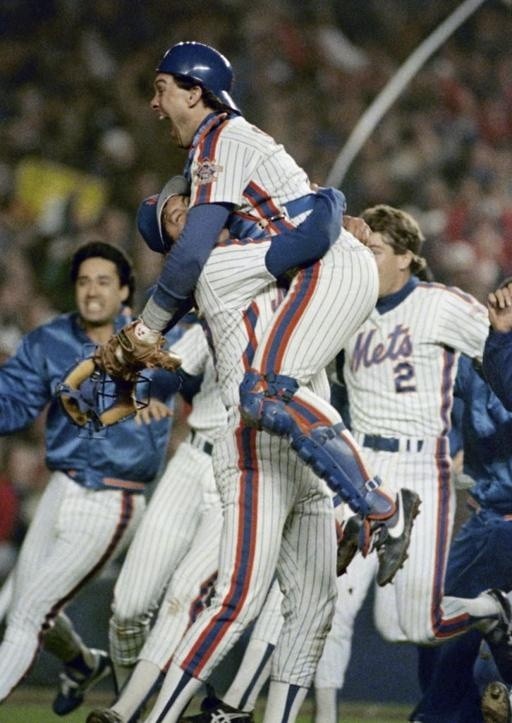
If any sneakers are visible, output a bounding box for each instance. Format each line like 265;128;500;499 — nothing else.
337;487;423;586
468;586;512;647
179;697;253;723
52;648;112;716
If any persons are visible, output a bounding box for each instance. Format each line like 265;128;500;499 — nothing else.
305;200;511;720
139;167;376;723
86;326;229;723
181;484;383;723
88;39;422;583
399;273;511;721
0;240;174;720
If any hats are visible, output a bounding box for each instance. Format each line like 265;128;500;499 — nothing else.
137;174;188;253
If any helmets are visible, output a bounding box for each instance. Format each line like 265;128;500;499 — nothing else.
58;356;152;432
72;241;132;284
156;41;240;113
